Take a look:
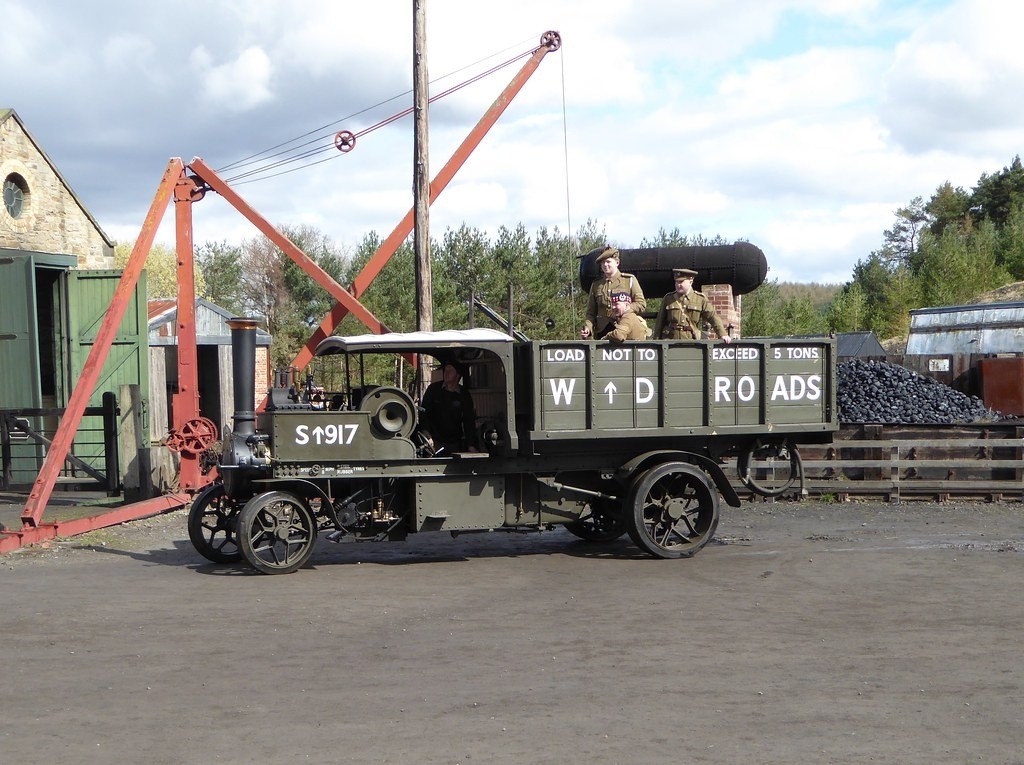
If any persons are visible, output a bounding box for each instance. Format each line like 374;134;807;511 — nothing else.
581;249;646;340
601;292;646;341
419;361;478;453
653;269;731;344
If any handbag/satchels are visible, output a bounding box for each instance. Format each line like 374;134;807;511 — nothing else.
691;324;702;340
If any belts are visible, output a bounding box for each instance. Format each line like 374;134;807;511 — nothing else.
668;323;692;331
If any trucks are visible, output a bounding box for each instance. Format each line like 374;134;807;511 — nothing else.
187;328;839;575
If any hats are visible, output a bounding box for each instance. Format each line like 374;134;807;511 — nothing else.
595;248;620;264
610;291;632;304
672;268;699;281
440;357;465;377
